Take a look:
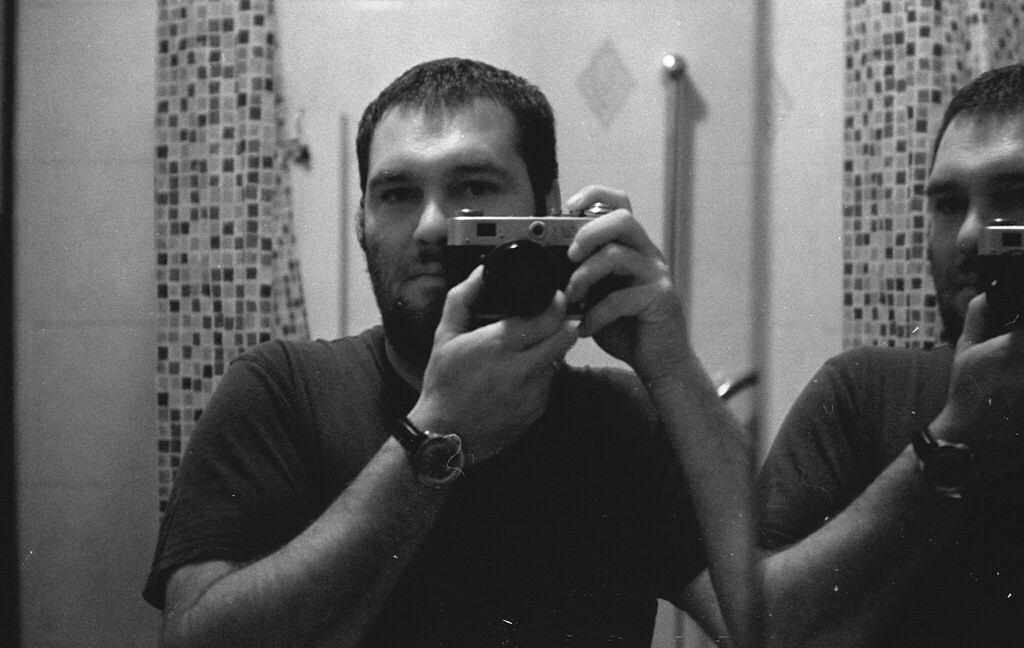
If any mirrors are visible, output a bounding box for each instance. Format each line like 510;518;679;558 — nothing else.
0;0;1024;648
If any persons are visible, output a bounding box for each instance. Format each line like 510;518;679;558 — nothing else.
757;65;1024;648
138;57;759;648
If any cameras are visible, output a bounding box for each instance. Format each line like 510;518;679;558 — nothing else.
978;218;1024;341
445;203;635;322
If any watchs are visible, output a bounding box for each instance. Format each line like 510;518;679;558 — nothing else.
910;424;984;501
392;416;473;490
